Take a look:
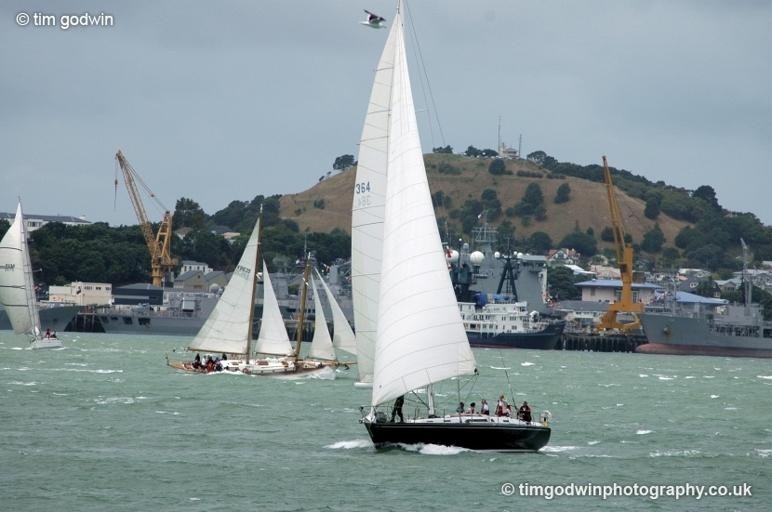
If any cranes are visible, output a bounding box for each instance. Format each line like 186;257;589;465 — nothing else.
596;155;646;332
113;148;179;287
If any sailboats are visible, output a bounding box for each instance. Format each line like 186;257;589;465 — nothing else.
351;0;553;453
165;202;355;376
0;194;64;351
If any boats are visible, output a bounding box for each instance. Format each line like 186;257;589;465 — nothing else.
457;301;566;350
0;300;81;332
634;236;772;359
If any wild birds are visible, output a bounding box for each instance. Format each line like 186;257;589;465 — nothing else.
302;277;311;288
361;9;385;27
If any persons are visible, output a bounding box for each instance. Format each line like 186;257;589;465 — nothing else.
388;395;405;423
190;352;227;373
480;399;490;416
465;402;479;414
44;327;50;340
518;401;531;415
516;405;532;423
494;394;512;417
456;402;465;414
